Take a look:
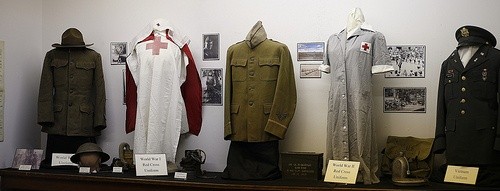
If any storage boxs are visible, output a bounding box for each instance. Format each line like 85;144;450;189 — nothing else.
280;152;323;182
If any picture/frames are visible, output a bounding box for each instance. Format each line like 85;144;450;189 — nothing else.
202;33;221;61
110;41;127;65
296;42;325;61
383;86;427;113
385;44;426;79
299;64;322;78
199;68;224;106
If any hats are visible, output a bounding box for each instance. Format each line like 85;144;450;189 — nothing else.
244;21;268;49
52;27;94;48
455;25;498;48
70;143;110;167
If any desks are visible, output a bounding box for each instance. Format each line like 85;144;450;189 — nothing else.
0;167;423;191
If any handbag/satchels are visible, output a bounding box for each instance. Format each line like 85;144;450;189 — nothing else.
380;134;434;178
